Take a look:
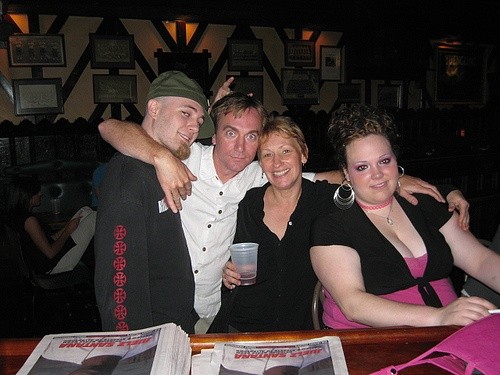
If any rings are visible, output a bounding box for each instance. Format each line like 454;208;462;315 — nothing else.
224;274;227;280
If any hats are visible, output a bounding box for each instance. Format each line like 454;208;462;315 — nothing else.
145;71;215;139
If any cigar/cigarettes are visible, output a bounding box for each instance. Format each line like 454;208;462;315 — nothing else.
488;309;500;314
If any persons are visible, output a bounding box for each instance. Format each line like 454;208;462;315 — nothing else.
309;103;500;329
99;92;445;335
95;71;253;334
0;174;94;289
222;116;470;332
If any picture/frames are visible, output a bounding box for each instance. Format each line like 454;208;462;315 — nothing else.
370;80;404;109
89;33;135;70
93;73;138;104
280;68;321;105
6;32;66;67
226;37;263;71
10;77;64;116
336;80;365;106
320;45;343;82
225;75;263;103
284;39;316;67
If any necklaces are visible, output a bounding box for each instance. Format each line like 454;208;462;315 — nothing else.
357;196;394;224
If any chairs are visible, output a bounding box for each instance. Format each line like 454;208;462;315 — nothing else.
0;224;101;336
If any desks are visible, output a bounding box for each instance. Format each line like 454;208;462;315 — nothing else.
1;325;500;375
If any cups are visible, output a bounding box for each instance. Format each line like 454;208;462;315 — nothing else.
50;198;62;215
228;242;259;287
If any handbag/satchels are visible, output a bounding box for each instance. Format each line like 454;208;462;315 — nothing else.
367;313;500;375
